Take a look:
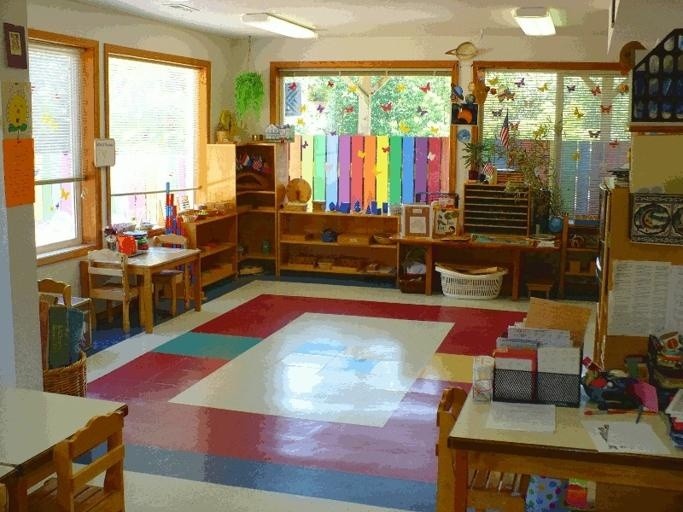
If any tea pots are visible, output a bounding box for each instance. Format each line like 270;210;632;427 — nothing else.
320;229;338;242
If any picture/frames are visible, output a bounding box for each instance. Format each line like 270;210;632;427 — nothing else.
3;22;28;69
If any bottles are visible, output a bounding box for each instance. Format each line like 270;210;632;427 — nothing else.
329;201;335;211
354;205;361;212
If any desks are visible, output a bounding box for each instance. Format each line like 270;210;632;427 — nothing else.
444;383;683;512
79;246;202;334
388;229;563;302
0;386;129;512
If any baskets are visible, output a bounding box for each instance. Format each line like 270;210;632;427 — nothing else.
493;347;583;408
434;265;510;300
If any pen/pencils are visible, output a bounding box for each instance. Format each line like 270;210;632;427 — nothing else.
635;407;643;423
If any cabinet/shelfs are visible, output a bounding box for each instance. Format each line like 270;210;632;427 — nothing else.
160;140;401;301
559;120;683;390
458;180;537;238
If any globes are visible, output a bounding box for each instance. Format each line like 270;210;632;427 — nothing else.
549;217;562;235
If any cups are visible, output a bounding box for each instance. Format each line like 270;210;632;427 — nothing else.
370;201;388;215
472;354;495;406
340;202;350;214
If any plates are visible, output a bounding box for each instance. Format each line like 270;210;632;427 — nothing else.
530;233;556;241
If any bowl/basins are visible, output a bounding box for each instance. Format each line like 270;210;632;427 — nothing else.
196;213;208;219
581;374;622;403
373;232;396;245
181;215;198;223
201;208;217;217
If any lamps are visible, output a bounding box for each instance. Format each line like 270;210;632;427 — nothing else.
241;12;319;41
510;6;557;37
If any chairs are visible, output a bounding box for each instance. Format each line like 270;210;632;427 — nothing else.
433;384;569;511
37;277;93;351
87;248;154;333
136;233;189;316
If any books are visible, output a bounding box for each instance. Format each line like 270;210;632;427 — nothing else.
493;323;580;406
